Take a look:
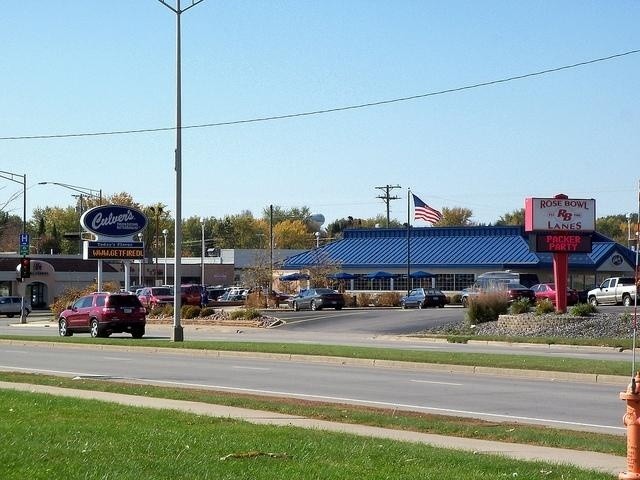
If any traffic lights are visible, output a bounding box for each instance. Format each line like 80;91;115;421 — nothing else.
21;259;31;278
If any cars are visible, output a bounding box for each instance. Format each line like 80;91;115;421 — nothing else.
398;270;579;310
134;284;345;313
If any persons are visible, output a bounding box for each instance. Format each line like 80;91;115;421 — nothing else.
200;287;209;310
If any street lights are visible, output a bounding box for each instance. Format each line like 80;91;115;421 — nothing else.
137;233;143;286
315;231;321;249
199;217;207;288
37;181;102;206
162;229;169;285
625;212;633;240
71;194;90;254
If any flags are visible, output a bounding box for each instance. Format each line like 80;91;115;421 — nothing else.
411;193;444;225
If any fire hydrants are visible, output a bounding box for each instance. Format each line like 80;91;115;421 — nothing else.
619;372;640;480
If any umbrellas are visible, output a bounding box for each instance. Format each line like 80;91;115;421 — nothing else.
277;272;314;293
325;272;361;293
408;270;437;287
363;270;399;292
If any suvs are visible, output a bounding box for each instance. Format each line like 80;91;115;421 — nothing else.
0;296;32;317
58;291;147;339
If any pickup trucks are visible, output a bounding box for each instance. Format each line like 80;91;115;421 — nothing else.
587;276;640;307
